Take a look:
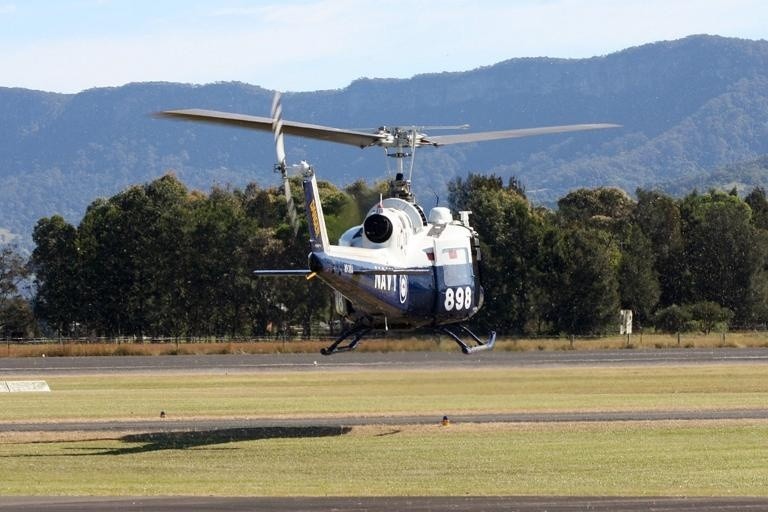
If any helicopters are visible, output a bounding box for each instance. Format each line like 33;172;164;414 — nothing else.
149;92;623;355
